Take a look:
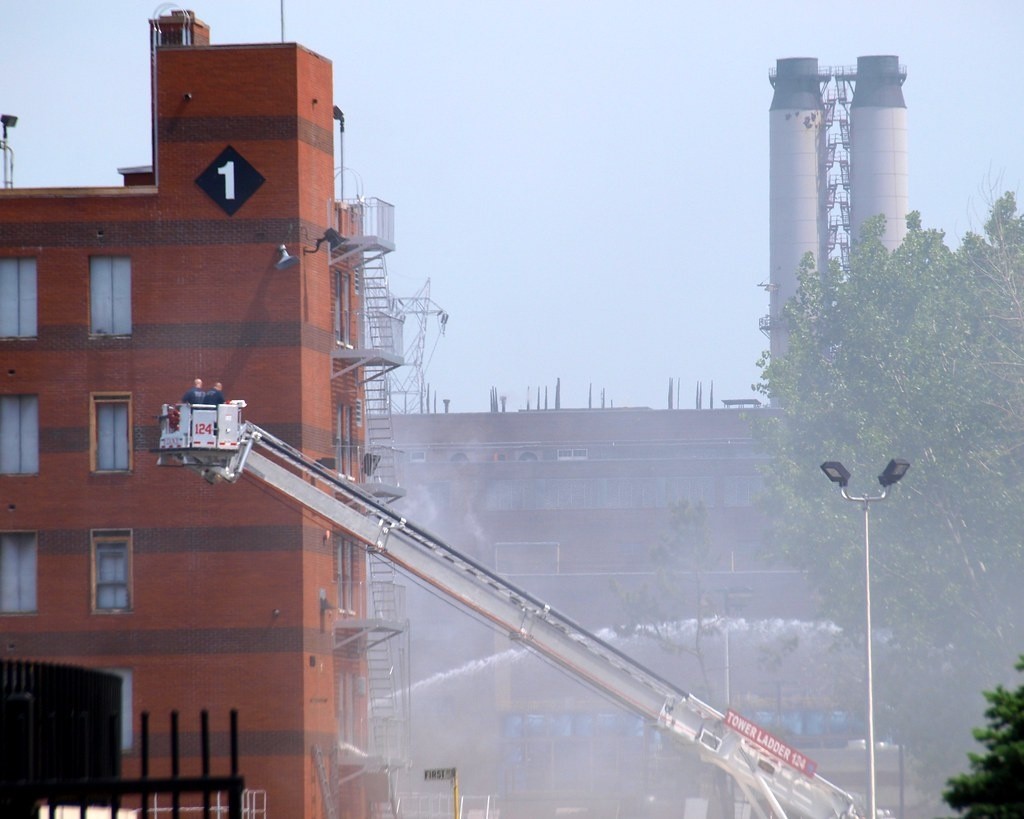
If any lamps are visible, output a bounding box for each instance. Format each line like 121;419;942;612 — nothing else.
303;227;350;256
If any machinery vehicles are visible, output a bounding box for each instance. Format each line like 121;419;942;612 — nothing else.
156;398;893;819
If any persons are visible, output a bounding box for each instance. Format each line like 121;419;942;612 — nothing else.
176;379;226;448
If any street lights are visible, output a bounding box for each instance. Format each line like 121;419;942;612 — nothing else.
0;114;17;189
332;107;345;201
820;460;911;819
